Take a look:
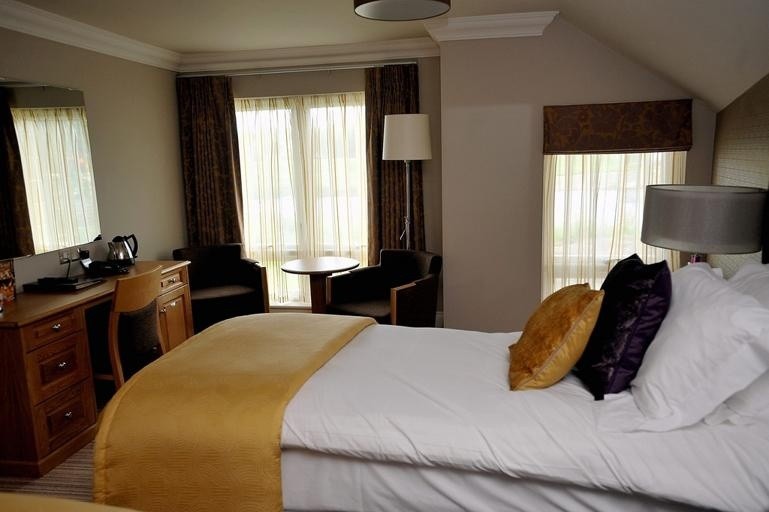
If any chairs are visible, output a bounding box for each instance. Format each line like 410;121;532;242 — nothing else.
171;243;270;336
325;248;442;328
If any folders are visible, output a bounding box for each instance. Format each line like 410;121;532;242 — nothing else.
22;279;104;291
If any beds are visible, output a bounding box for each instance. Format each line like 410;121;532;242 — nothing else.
93;311;769;512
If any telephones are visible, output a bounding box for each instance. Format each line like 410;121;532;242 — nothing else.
88;261;128;276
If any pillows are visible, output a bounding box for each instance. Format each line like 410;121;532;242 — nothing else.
700;265;769;428
629;262;769;434
570;254;672;402
509;281;606;392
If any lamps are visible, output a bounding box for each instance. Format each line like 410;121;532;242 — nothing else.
353;0;451;21
640;184;769;264
382;113;434;249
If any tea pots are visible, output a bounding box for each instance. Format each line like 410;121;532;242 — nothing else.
107;234;139;267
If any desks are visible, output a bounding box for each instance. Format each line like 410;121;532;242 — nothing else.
280;257;360;313
